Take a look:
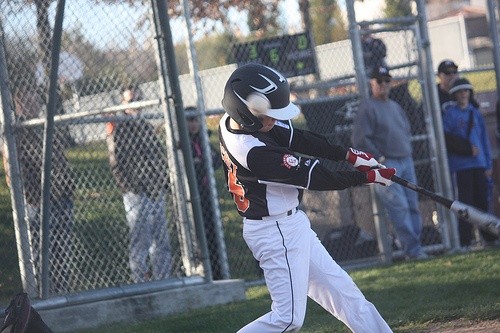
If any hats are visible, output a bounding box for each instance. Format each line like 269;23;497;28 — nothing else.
438;61;458;72
370;67;390;77
449;78;473;94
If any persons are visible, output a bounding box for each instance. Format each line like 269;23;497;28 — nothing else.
360;20;390;80
218;64;397;333
161;104;225;281
107;84;172;285
351;65;437;263
435;59;500;249
3;84;75;298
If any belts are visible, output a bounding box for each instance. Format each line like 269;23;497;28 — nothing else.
244;206;299;220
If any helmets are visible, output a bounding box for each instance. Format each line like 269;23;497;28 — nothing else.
222;63;301;131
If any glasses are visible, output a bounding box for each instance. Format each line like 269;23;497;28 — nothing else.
378;78;391;83
443;70;457;74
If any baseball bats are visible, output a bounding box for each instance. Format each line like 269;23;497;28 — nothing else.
374;164;500;233
